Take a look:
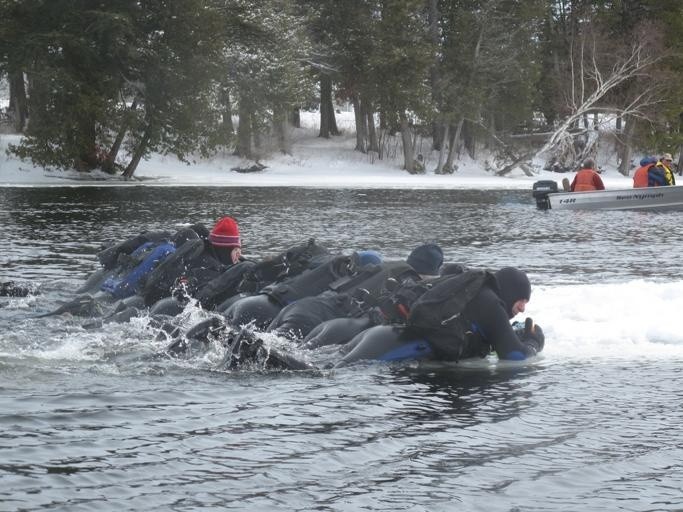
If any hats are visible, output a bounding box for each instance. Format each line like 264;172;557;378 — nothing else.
405;244;443;276
660;153;672;161
207;216;241;249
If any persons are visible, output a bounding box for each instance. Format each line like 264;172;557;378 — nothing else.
633;156;671;187
654;153;677;186
569;157;606;191
0;215;547;375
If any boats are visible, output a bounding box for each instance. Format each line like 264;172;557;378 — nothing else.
532;180;683;211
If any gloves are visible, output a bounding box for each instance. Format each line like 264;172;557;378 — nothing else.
522;317;545;353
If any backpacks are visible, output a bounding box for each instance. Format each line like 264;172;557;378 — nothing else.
408;266;501;330
148;237;205;293
